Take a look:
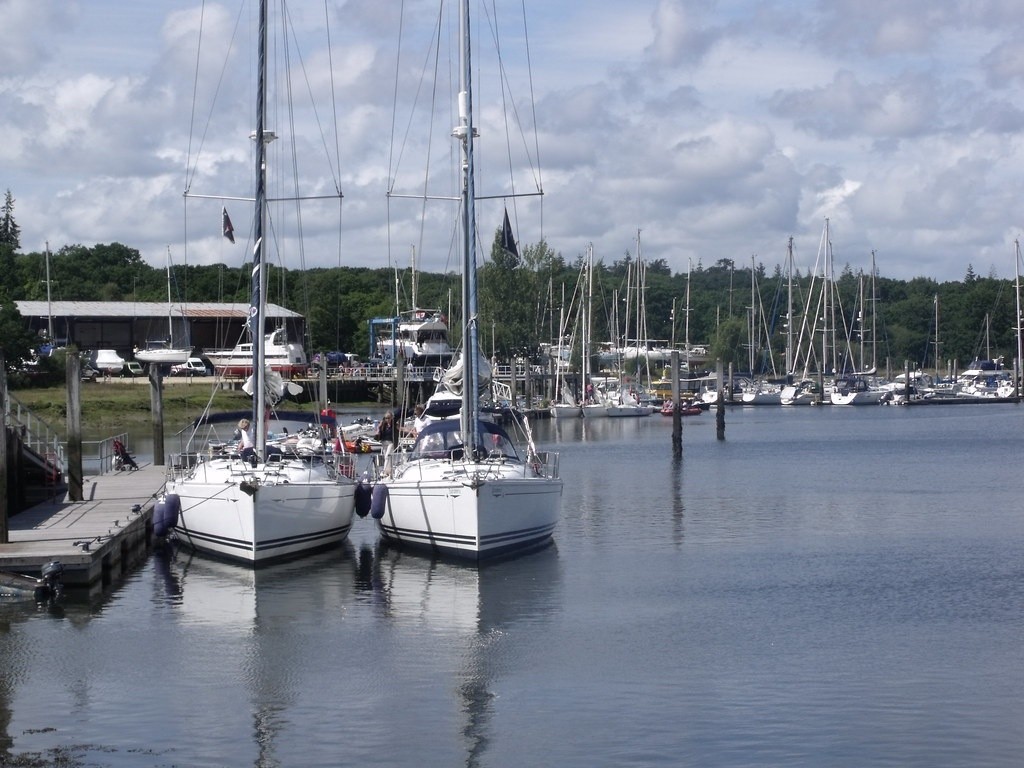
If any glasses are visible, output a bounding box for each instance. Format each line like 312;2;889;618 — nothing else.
385;418;390;419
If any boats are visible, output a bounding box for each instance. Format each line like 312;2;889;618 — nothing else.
546;215;1023;418
204;416;416;461
155;0;359;572
373;244;454;369
134;246;192;366
201;130;312;381
81;348;125;375
369;1;565;568
20;242;66;369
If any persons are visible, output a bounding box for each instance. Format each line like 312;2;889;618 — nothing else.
237;418;254;450
412;405;432;447
407;362;413;372
339;364;343;372
378;412;419;477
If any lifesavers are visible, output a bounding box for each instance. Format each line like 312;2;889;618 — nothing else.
321;408;338;440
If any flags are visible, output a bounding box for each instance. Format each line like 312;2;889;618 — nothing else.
500;209;519;260
222;209;236;243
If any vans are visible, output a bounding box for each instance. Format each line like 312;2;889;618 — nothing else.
171;357;206;376
123;361;144;376
